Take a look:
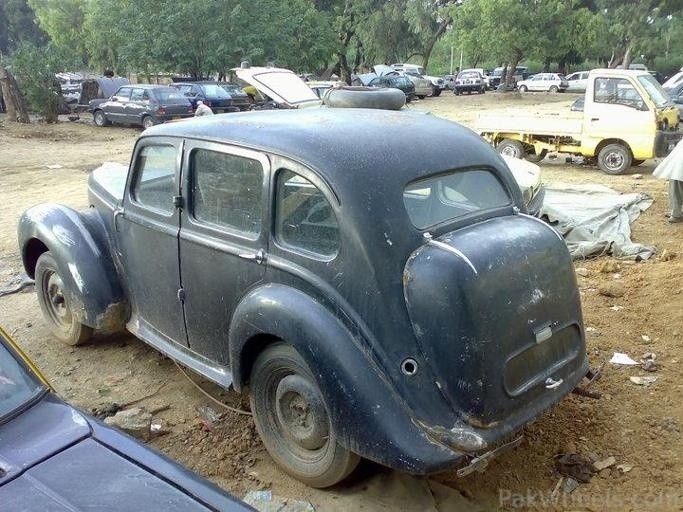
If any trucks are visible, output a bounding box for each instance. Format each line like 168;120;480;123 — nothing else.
481;70;682;174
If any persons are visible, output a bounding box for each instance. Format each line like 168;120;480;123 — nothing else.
194;100;214;117
653;140;683;222
97;70;114;98
329;65;369;86
455;67;460;78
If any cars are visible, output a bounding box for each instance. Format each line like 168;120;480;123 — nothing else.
661;68;682;122
17;107;590;488
234;67;544;220
0;326;256;511
